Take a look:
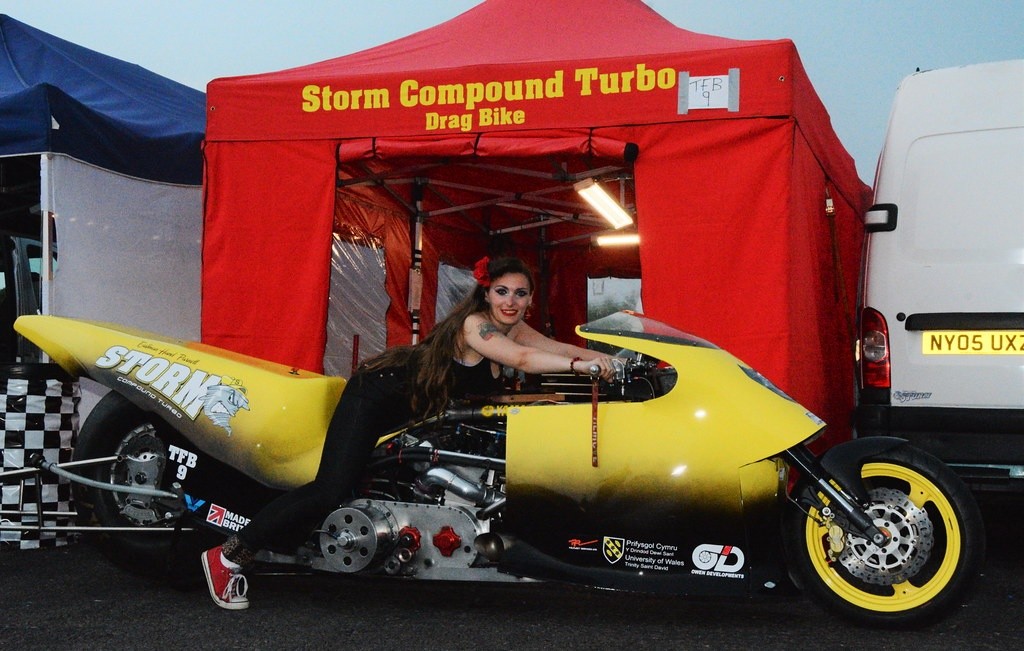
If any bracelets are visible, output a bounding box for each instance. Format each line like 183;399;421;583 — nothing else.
570;356;583;378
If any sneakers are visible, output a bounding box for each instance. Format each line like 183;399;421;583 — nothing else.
201;543;250;611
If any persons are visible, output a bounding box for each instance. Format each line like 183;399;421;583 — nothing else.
196;254;630;611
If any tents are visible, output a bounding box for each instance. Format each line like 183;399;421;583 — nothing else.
202;2;875;509
1;12;205;379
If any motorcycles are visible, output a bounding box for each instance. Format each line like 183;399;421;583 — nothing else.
13;305;992;635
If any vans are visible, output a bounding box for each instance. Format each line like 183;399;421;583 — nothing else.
848;61;1024;496
